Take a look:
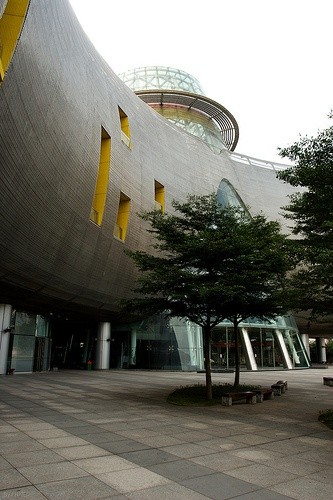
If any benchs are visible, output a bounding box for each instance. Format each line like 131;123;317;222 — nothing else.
322;376;333;388
221;380;288;407
